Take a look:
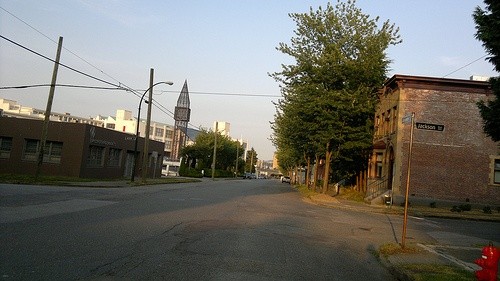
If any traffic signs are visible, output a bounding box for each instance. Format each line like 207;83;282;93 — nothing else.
402;115;412;124
416;122;444;132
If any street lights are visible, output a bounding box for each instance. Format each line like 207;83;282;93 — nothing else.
131;81;173;182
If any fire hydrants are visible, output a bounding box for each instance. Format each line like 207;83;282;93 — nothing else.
474;240;500;281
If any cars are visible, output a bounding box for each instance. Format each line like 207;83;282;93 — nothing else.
281;176;291;184
243;172;267;180
